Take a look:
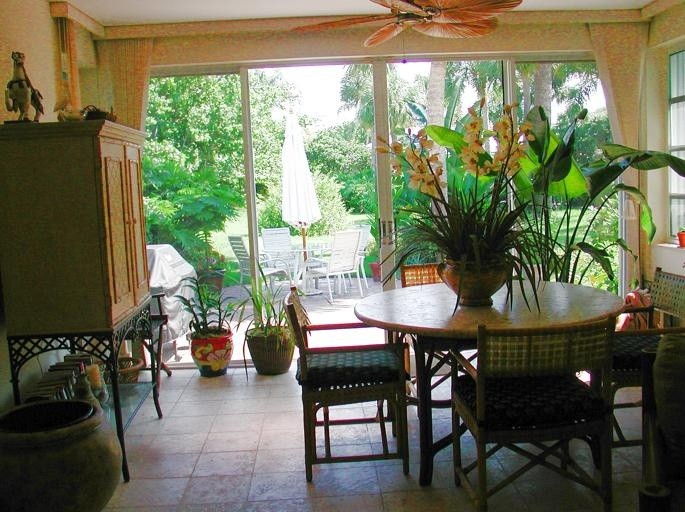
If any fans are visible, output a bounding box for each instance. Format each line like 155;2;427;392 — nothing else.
282;0;527;53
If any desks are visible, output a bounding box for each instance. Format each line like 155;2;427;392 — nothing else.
5;294;165;485
353;281;624;487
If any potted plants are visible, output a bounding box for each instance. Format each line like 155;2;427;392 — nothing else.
215;255;305;384
677;225;685;248
173;268;243;378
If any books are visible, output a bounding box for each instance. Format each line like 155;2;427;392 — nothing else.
22;351;95;402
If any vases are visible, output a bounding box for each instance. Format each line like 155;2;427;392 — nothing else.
434;252;516;309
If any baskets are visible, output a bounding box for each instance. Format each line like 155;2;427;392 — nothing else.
92;355;141;397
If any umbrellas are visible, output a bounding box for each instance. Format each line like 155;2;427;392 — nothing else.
279;105;323;269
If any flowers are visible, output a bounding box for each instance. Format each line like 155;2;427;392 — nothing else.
368;96;576;319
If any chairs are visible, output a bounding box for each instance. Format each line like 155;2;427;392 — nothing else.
451;313;621;510
398;258;451;401
97;291;172;391
600;264;683;452
283;283;413;487
229;225;372;304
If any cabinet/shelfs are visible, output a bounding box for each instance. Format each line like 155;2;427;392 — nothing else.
1;121;151;334
